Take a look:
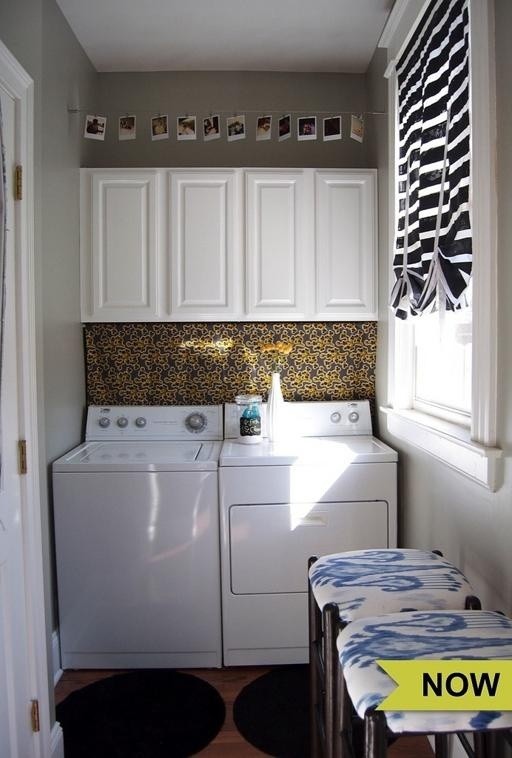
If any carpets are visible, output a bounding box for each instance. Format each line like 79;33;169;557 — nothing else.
55;665;310;758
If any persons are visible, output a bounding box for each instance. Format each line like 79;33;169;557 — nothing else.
87;118;104;134
154;120;167;135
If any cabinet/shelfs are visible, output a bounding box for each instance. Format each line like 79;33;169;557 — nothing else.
308;548;512;757
77;165;379;322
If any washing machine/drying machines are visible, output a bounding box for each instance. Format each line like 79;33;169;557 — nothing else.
218;398;400;666
52;403;224;669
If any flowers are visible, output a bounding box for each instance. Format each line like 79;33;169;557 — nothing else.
262;342;292;371
